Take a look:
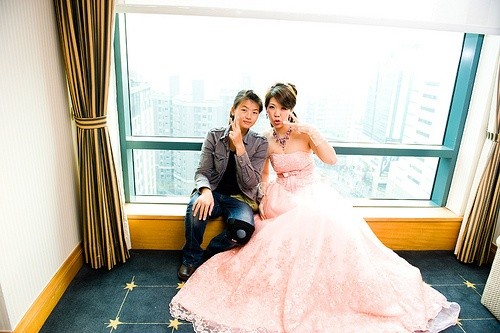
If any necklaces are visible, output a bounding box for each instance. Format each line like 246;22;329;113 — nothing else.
272;126;293;150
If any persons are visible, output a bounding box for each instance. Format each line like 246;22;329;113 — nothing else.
177;89;269;281
258;78;337;333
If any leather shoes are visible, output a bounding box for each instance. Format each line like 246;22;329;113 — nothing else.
177;262;194;281
